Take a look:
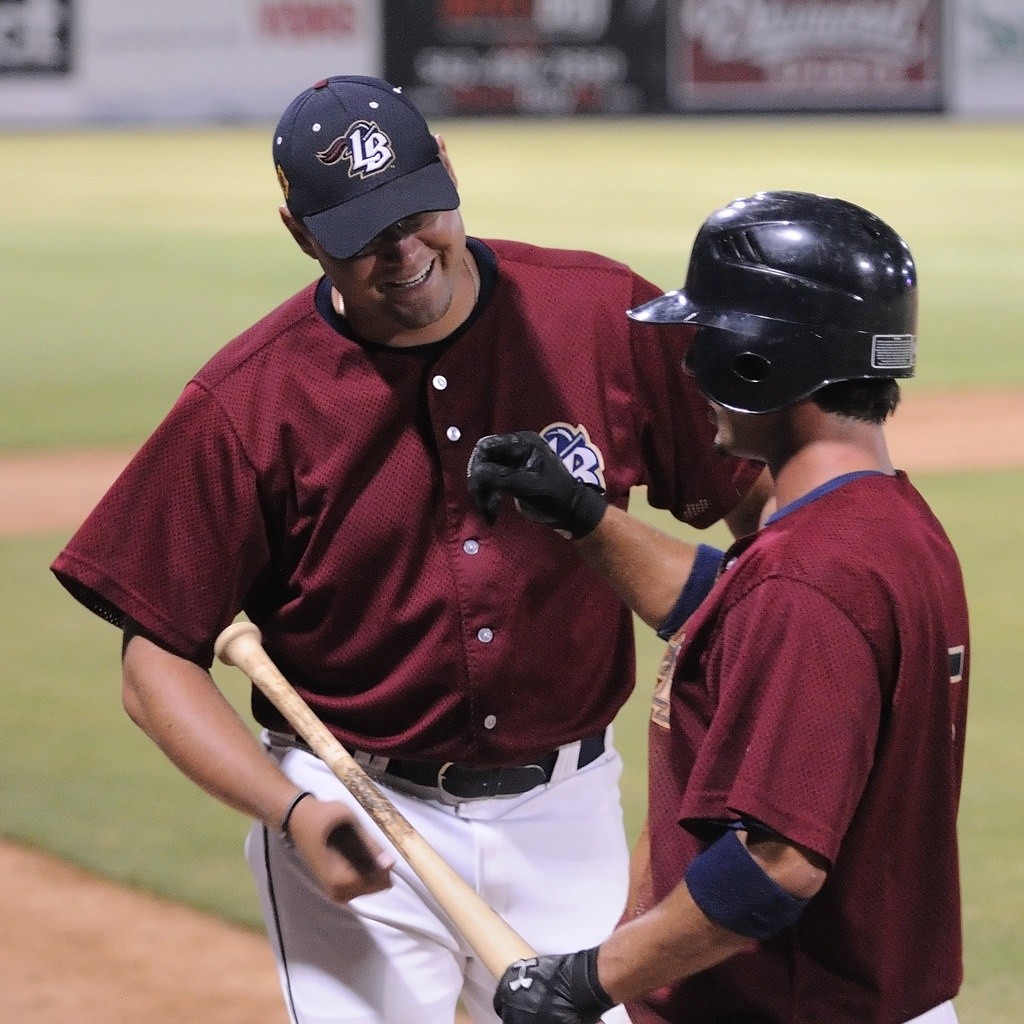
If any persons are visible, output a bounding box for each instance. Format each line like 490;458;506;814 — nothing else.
466;190;972;1024
51;75;776;1024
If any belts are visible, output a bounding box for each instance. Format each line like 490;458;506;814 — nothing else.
297;730;608;798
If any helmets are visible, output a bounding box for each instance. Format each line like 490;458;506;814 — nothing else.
623;190;920;417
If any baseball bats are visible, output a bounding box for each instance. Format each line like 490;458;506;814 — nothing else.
213;621;607;1024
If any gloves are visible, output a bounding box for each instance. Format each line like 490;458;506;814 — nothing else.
493;944;618;1024
465;429;607;539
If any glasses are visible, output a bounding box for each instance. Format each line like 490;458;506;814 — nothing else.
355;209;441;257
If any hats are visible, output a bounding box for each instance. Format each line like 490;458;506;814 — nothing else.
271;73;462;261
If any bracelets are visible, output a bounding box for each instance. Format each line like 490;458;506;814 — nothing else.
280;793;311;849
